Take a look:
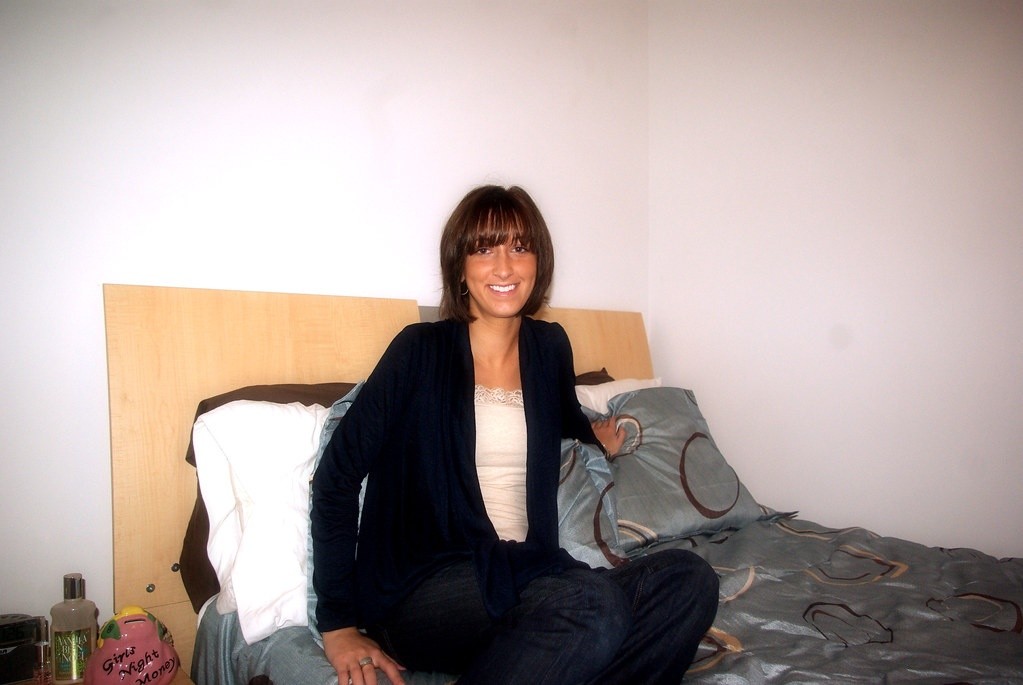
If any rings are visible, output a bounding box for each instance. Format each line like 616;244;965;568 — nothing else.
359;656;372;667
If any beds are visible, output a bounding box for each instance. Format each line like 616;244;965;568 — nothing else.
104;283;1023;685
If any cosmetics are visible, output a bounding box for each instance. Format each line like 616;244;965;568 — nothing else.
47;572;97;685
31;641;54;685
0;613;47;683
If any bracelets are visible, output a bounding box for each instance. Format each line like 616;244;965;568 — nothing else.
602;443;610;460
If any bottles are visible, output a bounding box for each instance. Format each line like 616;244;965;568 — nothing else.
50;572;97;685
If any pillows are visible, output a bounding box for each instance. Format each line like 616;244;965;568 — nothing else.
558;437;631;568
574;377;798;558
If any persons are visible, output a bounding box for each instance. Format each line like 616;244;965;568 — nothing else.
310;184;720;685
